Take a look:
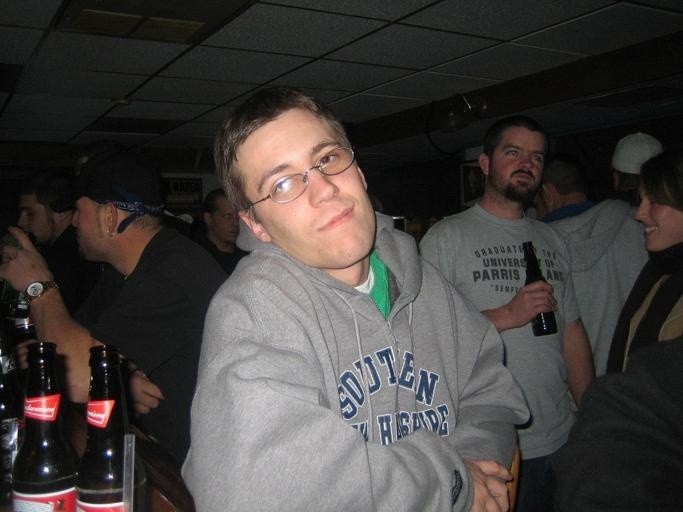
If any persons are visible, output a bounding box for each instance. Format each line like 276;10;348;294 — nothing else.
419;113;597;467
179;88;533;511
202;187;251;276
0;148;231;465
603;144;683;511
538;150;652;378
610;132;663;206
17;173;110;317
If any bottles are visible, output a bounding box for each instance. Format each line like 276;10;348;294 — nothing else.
13;293;35;341
522;239;558;337
11;343;79;512
77;346;147;511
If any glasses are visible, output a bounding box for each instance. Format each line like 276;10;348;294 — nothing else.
244;146;355;210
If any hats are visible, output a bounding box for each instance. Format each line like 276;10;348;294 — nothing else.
47;148;164;204
611;131;663;175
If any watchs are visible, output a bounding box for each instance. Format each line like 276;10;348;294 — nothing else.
22;279;60;303
